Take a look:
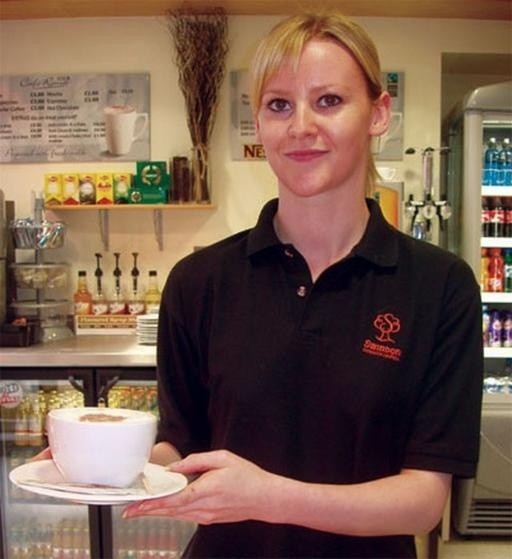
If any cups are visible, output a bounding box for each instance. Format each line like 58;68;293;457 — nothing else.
103;103;148;155
48;408;157;488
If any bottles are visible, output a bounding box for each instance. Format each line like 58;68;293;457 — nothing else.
489;312;502;346
136;161;170;203
504;197;512;236
482;197;490;235
15;389;84;445
490;197;505;236
97;385;159;414
484;137;500;185
74;271;91;315
499;138;512;186
112;521;192;559
503;248;512;291
8;447;43;500
8;517;90;559
489;248;503;291
481;248;488;290
145;270;162;313
503;313;512;346
482;305;491;345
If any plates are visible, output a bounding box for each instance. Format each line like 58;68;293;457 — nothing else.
9;460;189;504
138;315;161;345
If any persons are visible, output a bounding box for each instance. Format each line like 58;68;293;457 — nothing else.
26;10;484;555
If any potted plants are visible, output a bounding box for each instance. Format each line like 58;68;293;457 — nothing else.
161;3;230;205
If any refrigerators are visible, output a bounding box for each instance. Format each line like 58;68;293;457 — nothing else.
442;83;512;535
